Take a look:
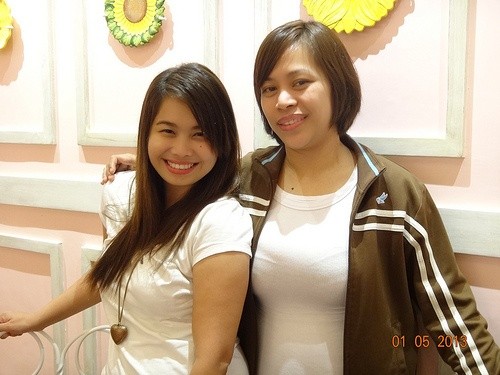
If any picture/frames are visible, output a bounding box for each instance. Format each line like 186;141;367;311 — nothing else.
77;0;217;147
253;0;465;157
0;0;55;144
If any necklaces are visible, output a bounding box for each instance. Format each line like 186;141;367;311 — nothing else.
110;240;156;346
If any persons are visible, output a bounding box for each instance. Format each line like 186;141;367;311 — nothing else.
101;21;500;375
0;63;254;375
